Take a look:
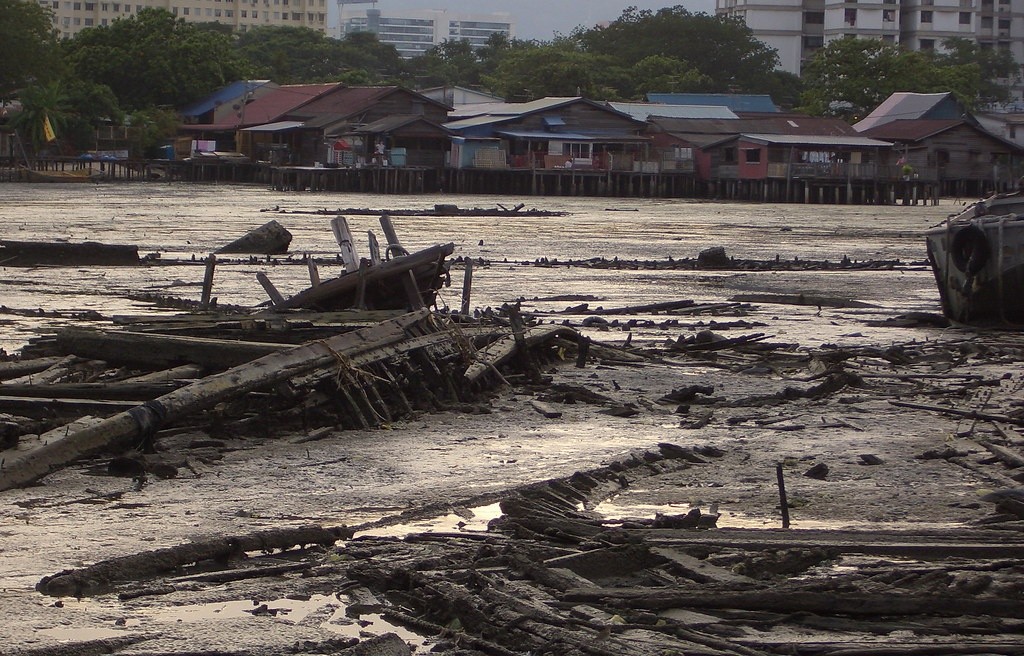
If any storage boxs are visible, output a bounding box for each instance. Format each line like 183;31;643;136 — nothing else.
191;140;215;151
390;147;406;167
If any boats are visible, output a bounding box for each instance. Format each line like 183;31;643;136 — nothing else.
924;189;1024;327
256;241;456;312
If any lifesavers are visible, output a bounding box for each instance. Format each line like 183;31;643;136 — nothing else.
950;224;990;274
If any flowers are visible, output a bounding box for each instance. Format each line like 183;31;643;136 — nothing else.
914;168;921;174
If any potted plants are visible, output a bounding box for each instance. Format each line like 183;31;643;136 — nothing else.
902;165;911;181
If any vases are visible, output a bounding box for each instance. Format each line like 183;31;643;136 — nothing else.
914;174;919;179
